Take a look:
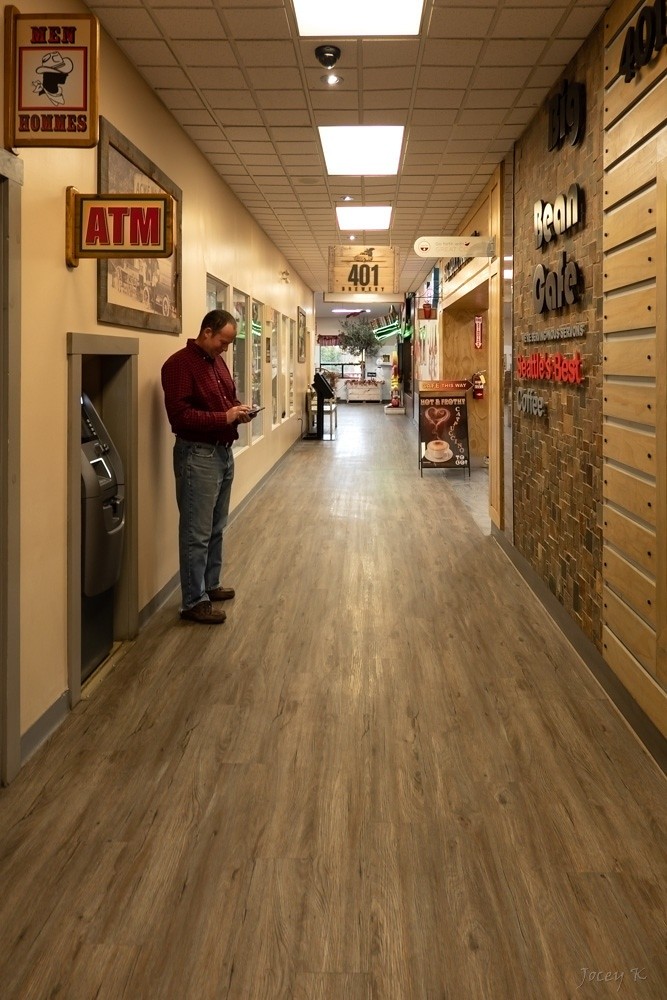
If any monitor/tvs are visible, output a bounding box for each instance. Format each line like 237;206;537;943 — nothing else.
81;406;98;444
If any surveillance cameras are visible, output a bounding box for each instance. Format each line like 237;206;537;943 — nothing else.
315;46;341;69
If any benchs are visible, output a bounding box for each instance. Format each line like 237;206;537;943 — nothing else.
307;389;338;442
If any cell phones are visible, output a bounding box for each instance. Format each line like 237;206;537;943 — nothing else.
247;406;264;414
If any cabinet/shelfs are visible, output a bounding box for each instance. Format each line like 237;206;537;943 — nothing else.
346;380;385;404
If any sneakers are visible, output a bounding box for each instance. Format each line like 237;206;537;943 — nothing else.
181;601;227;624
207;588;235;601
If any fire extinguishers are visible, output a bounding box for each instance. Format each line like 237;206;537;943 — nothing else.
471;369;487;400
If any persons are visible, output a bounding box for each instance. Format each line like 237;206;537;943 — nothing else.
160;309;258;624
